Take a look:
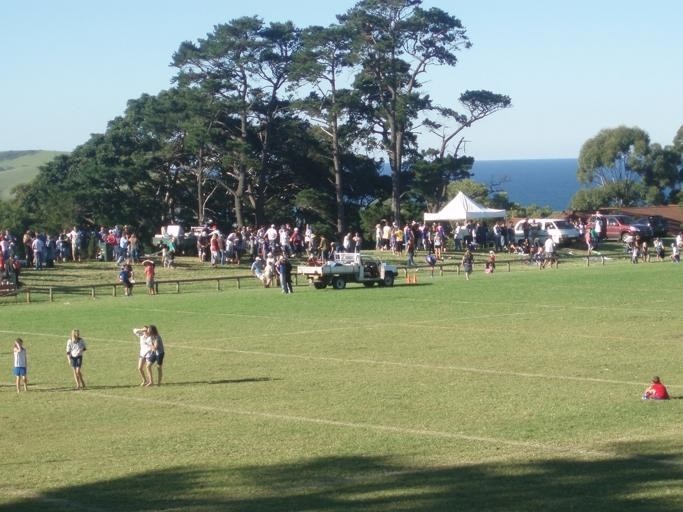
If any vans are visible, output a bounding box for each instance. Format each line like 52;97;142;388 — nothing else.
514;218;579;247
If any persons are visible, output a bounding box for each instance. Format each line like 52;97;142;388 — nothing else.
0;223;139;280
141;258;156;295
624;233;682;262
143;324;163;387
573;209;606;258
132;325;151;386
641;376;670;399
122;258;132;296
12;338;28;393
160;237;177;264
118;266;131;296
66;329;86;390
162;234;177;270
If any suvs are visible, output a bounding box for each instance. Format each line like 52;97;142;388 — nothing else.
585;215;662;242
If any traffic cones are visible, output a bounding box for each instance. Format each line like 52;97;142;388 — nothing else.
406;273;417;284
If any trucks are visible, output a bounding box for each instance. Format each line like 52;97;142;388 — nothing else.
296;252;400;291
152;224;224;256
